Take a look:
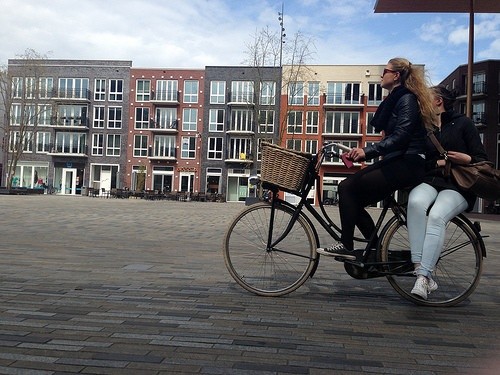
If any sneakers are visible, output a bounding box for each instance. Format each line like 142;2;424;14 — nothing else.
316;242;356;260
411;278;428;300
414;267;438;292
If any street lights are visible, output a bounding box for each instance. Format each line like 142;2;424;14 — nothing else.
271;1;286;147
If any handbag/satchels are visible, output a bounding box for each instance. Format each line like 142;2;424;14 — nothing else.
449;157;500;202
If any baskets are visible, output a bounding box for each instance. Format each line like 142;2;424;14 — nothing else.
260;141;312;191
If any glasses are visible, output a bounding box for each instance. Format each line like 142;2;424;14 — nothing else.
383;68;396;76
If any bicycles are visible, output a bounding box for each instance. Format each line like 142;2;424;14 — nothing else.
222;139;489;308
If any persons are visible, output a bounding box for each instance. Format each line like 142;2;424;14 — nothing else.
407;85;489;303
315;57;440;261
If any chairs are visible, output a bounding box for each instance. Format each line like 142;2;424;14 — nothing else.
85;187;227;204
